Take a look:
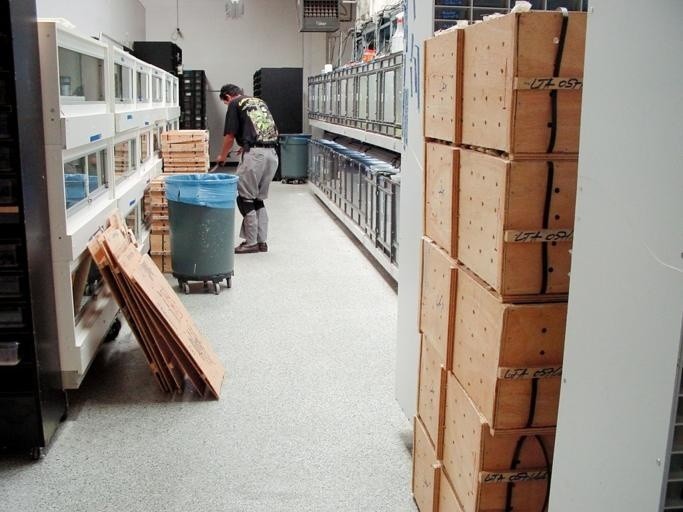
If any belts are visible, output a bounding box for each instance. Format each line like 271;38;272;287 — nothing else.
251;143;274;148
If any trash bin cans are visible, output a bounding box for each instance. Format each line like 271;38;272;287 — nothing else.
163;173;238;294
278;133;312;184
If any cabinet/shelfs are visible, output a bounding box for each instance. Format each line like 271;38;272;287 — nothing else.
304;0;401;284
0;0;183;462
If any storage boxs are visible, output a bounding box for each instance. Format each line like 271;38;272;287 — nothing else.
421;140;461;258
411;417;439;512
418;235;457;372
435;467;463;512
451;263;568;431
417;331;449;459
444;370;558;512
461;8;586;160
423;28;463;145
458;147;578;302
149;68;213;271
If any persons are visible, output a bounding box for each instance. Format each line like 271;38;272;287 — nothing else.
217;83;279;253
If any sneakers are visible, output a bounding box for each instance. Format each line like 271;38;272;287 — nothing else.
234;240;268;254
